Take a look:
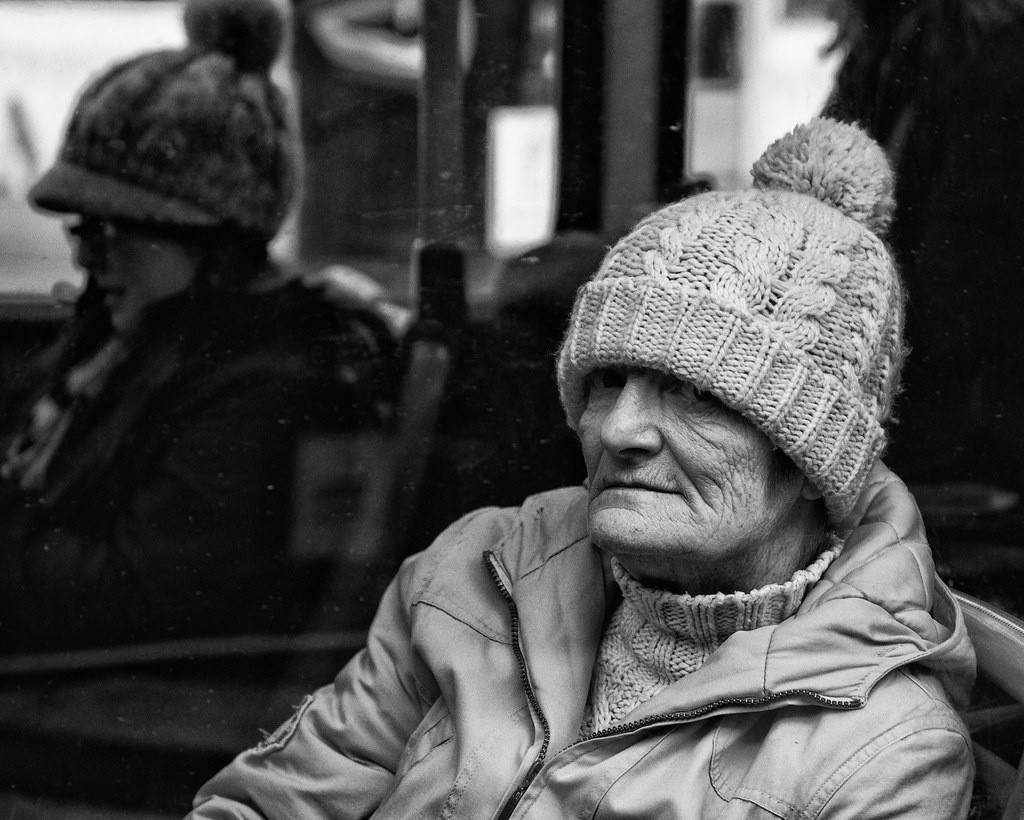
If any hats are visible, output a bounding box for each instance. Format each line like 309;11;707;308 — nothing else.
556;117;905;527
27;0;300;240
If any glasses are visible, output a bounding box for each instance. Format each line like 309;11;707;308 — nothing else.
65;222;130;247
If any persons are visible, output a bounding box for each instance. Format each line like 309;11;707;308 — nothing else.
0;1;401;688
182;122;977;820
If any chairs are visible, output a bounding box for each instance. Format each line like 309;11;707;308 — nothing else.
0;263;1024;820
